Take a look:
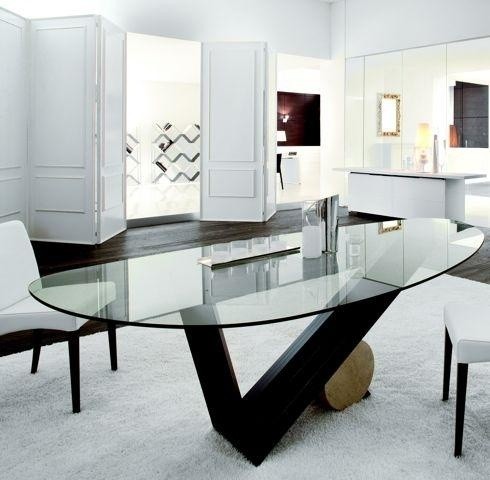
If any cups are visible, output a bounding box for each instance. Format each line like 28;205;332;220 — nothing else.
210;233;287;262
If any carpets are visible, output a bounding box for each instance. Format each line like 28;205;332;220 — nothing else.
1;267;490;479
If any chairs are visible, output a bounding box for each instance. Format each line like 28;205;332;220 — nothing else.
0;221;118;413
442;309;489;458
276;154;284;190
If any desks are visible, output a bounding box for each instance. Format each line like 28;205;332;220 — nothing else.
345;168;486;226
29;216;483;465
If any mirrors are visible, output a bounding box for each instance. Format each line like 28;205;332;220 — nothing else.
378;93;400;136
